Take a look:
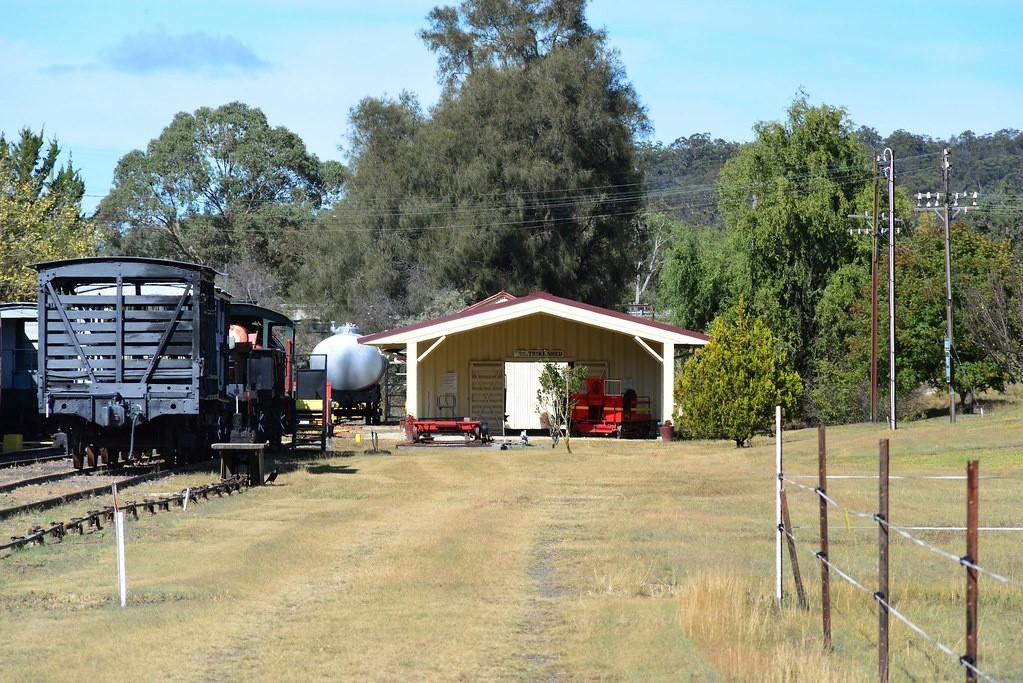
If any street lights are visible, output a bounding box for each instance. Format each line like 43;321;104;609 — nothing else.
875;146;902;428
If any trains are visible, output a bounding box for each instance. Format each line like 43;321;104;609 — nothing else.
308;322;388;426
26;255;302;470
